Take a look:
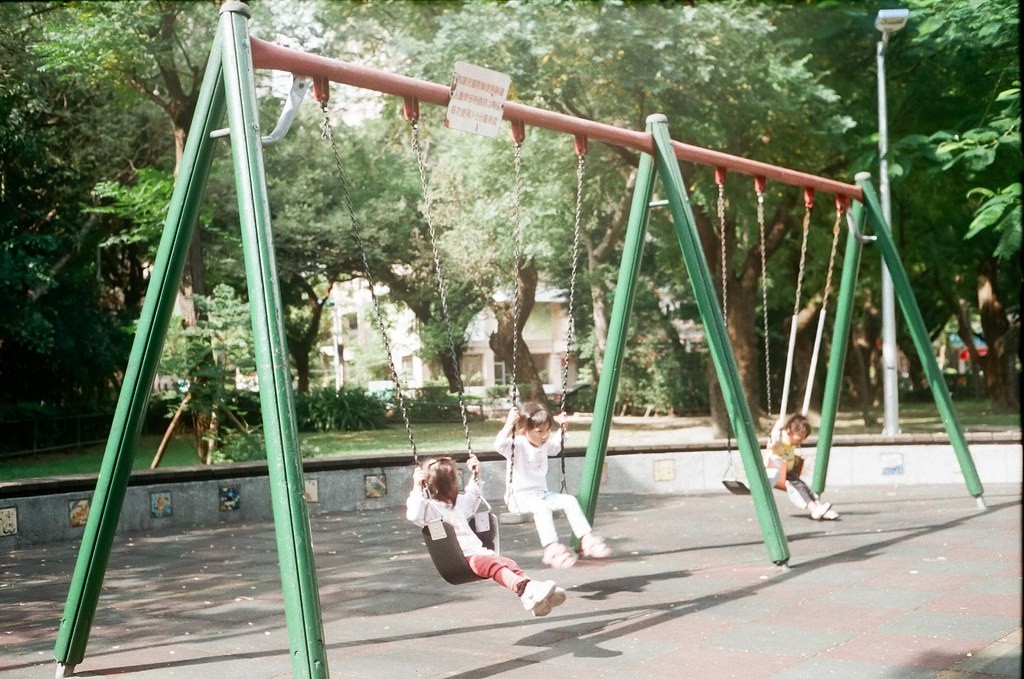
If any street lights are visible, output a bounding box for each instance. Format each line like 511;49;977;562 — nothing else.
874;8;911;434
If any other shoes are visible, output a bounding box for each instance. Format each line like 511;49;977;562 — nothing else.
823;510;838;519
812;502;832;519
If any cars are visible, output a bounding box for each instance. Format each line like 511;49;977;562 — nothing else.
557;382;596;414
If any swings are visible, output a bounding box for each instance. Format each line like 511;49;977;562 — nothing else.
318;106;501;585
502;146;571;525
709;184;781;494
766;204;849;490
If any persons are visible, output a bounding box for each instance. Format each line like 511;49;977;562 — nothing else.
765;414;839;518
495;403;613;569
406;455;565;616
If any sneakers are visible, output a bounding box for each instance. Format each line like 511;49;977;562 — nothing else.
542;544;577;568
532;587;566;616
582;536;613;558
521;580;556;611
